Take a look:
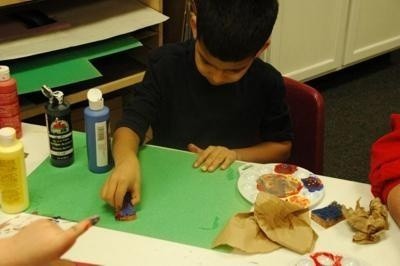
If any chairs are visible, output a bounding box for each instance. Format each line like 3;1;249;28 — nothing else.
281;76;325;175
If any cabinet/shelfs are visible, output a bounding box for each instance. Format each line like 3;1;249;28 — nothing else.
259;0;400;83
0;0;164;120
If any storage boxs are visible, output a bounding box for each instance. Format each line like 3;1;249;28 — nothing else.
70;97;123;132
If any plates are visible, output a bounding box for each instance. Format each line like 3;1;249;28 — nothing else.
237;161;325;210
293;252;360;266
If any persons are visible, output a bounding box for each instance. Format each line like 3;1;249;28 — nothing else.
367;113;400;229
101;0;295;212
0;215;101;265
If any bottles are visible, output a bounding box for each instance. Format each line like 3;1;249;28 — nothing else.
1;125;31;216
40;84;75;169
1;65;23;140
83;88;115;175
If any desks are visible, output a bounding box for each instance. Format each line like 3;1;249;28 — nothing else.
0;121;400;266
